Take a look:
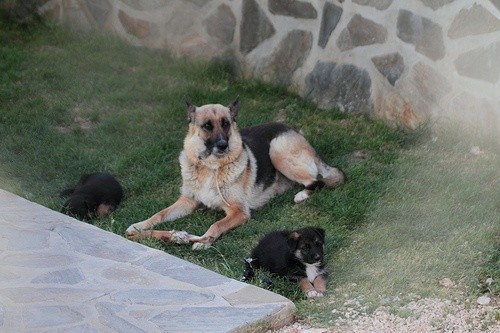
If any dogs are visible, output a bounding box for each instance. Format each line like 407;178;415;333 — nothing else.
60;172;122;224
244;226;328;298
125;95;348;252
